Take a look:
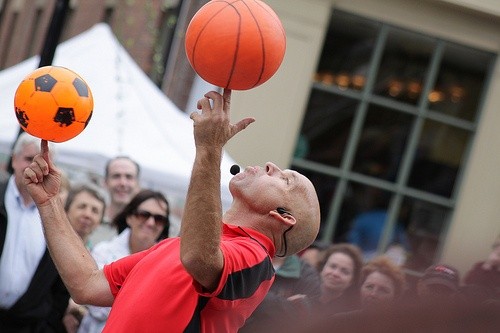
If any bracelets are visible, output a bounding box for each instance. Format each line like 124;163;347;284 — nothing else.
79;306;85;314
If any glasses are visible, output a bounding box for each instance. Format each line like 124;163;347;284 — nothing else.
132;209;167;225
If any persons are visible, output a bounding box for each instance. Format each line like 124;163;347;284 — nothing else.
299;245;324;268
417;262;461;305
410;207;438;272
0;133;70;333
77;188;170;333
22;89;319;333
237;253;320;333
347;196;412;264
355;257;409;312
467;233;500;298
62;180;110;333
89;155;144;246
317;244;363;313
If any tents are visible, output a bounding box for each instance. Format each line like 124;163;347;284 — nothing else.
0;19;244;219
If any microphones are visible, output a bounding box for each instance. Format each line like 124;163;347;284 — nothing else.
230;164;240;175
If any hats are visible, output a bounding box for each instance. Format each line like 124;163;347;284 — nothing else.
426;263;459;289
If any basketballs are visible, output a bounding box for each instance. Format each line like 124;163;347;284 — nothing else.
184;0;287;90
14;66;94;144
